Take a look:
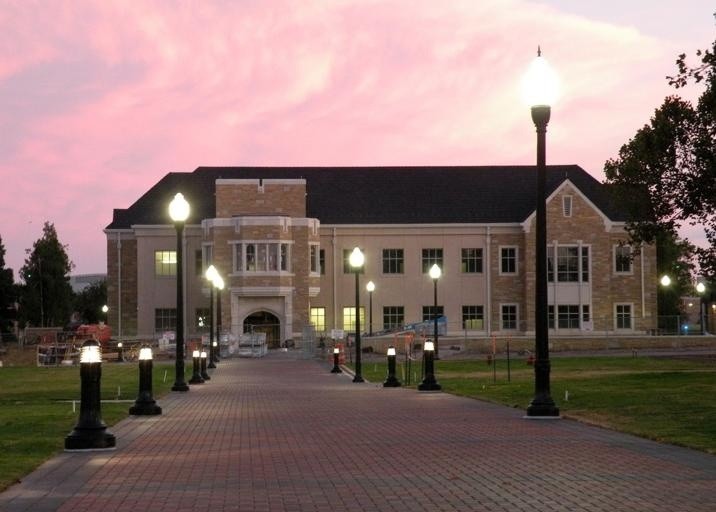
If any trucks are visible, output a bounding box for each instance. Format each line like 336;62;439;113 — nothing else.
239;331;268;358
158;331;186;359
200;330;236;359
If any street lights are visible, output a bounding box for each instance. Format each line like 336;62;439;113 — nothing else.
428;262;444;361
696;282;709;335
102;305;108;325
348;247;366;381
164;187;193;391
660;274;674;335
205;265;227;368
364;280;379;337
524;43;565;417
